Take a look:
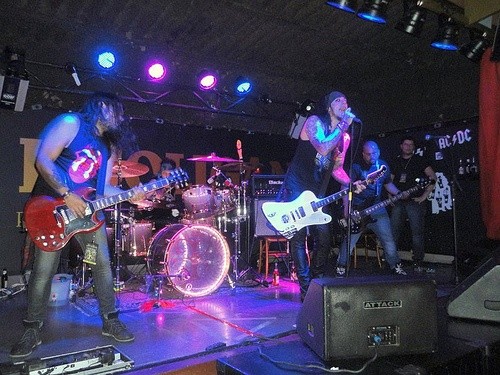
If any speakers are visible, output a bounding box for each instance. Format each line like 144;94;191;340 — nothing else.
295;246;500;363
251;197;283;237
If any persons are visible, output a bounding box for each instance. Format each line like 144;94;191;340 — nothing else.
9;96;148;358
278;90;366;303
386;135;437;269
137;156;180;234
207;166;231;191
335;139;409;275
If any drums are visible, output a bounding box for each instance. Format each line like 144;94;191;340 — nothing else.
105;186;236;298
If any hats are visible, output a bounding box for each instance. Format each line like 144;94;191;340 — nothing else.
325;91;346;108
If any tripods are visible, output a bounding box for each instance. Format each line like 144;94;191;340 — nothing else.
78;165;145;310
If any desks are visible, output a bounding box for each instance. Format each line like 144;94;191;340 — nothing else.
260;236;310;280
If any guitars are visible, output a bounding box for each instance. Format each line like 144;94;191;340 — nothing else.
23;166;191;252
260;164;388;241
335;178;436;235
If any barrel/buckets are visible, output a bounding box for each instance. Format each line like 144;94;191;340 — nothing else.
47;274;72;306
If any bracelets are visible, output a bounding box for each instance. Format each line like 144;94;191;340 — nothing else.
396;192;401;195
422;195;426;199
62;190;72;198
336;120;350;132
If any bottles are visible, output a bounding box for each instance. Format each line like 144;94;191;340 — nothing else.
272;263;279;286
290;265;296;281
1;269;8;291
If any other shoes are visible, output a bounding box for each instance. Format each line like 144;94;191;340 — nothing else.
414;262;422;272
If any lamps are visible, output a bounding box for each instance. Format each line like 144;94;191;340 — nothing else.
324;0;358;15
395;6;426;36
461;31;490;61
356;0;392;24
431;16;459;51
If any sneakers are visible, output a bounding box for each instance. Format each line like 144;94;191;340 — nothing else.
102;309;136;342
391;263;408;275
335;266;347;279
9;320;45;358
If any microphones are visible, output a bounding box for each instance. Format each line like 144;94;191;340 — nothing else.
354;118;362;124
148;207;179;217
207;175;217;185
183;273;190;279
425;135;451;140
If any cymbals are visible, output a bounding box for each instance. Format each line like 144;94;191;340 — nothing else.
186;156;239;162
110;160;150;178
